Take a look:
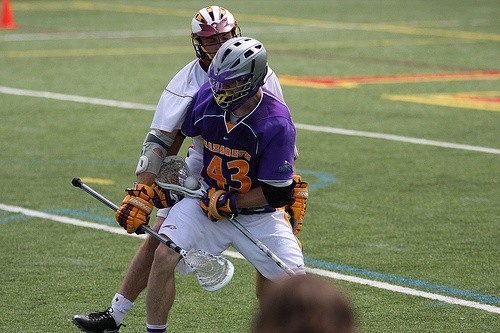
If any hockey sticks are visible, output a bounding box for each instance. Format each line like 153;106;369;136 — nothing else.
72;176;235;292
154;155;297;277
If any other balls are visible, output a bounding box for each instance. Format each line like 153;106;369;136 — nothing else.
183;176;199;190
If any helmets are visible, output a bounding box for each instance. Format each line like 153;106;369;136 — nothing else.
208;37;267;113
192;5;236;69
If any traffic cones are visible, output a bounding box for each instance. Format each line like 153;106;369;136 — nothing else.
0;0;17;31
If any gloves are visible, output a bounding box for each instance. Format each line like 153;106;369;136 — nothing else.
114;184;154;235
199;187;239;223
281;175;308;234
153;176;183;208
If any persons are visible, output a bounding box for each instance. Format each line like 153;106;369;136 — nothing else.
70;6;309;333
251;273;355;333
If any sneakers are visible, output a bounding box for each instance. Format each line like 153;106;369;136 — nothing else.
72;307;126;333
